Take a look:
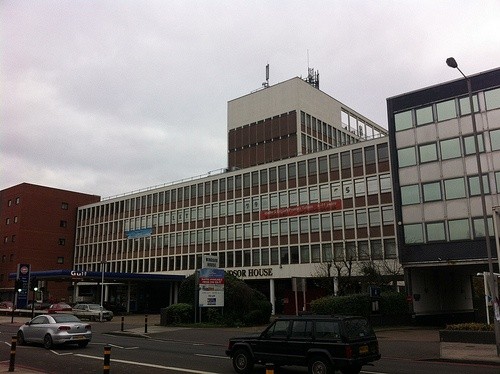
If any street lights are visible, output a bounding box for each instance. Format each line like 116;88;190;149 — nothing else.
446;57;500;356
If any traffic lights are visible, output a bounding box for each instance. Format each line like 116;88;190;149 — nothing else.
31;276;39;291
16;280;23;293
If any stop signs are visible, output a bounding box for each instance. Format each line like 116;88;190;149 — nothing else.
405;295;412;303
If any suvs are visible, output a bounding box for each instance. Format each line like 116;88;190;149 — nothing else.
225;315;382;374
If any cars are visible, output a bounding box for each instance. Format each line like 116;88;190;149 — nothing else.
17;314;92;350
26;300;48;310
48;303;72;314
0;301;13;314
72;304;113;321
